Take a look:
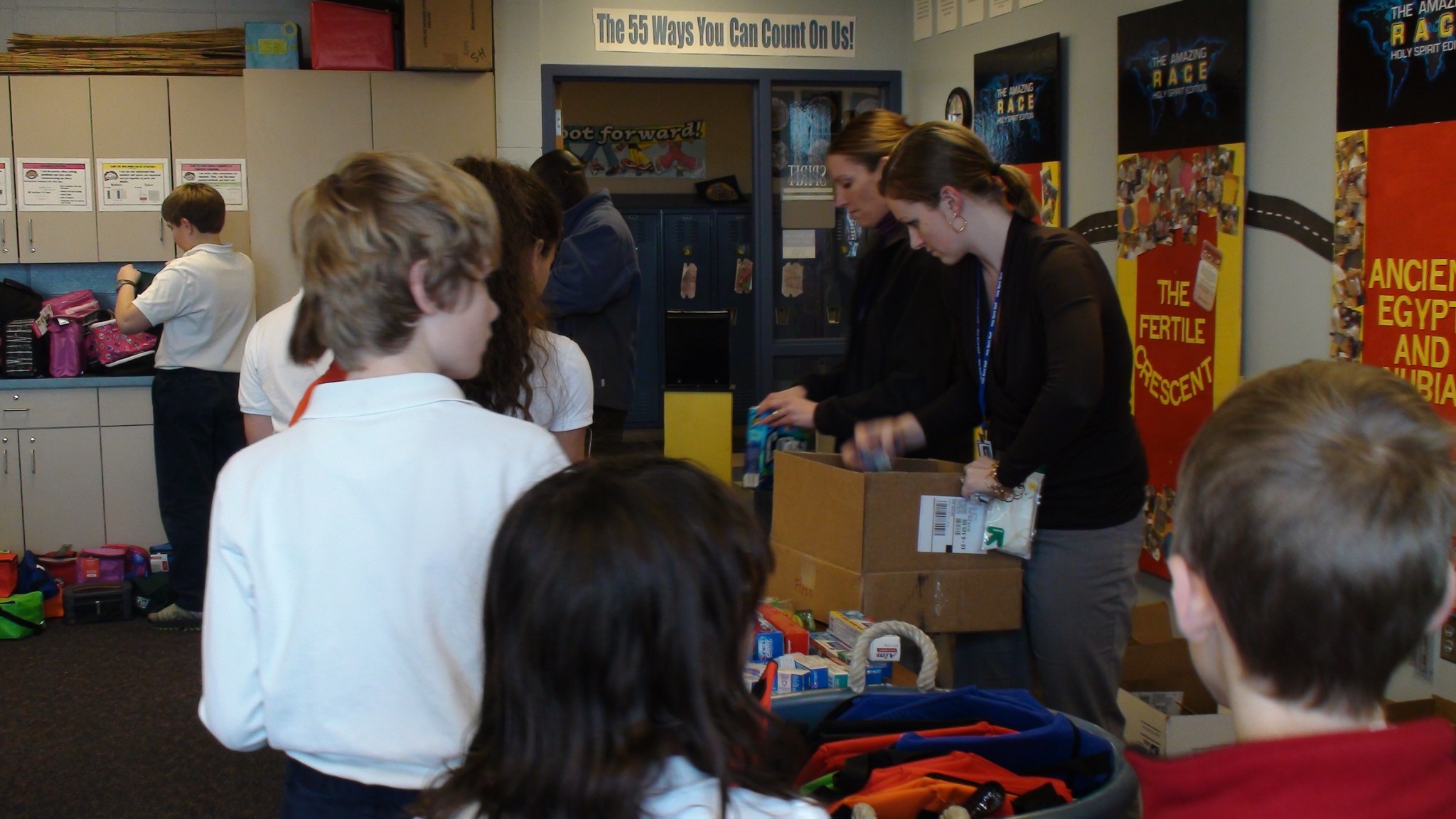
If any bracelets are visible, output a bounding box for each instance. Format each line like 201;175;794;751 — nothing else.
985;462;1006;500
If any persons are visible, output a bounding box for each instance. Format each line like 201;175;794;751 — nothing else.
1116;147;1237;257
755;111;975;456
845;121;1147;741
195;153;571;819
449;156;593;464
528;149;642;460
413;452;829;819
1129;363;1456;819
1334;141;1365;360
239;289;335;442
115;182;256;628
984;448;988;456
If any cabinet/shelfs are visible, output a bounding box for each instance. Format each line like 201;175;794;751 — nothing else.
0;75;99;264
98;386;169;554
0;388;107;563
89;73;249;262
244;67;497;322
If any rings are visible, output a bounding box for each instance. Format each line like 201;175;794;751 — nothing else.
773;411;780;418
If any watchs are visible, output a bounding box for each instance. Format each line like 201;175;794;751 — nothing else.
116;279;136;293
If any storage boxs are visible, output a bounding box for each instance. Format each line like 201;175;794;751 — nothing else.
744;449;1023;698
1113;603;1236;756
403;0;494;71
310;0;394;70
243;21;302;69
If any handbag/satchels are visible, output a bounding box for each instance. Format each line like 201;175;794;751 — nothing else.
41;289;101;325
89;310;157;367
85;334;99;364
1;541;179;639
50;315;82;377
0;277;43;325
742;620;1139;818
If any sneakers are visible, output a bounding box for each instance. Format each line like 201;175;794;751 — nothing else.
147;603;202;633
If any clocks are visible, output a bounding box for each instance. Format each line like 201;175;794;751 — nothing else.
944;87;972;130
